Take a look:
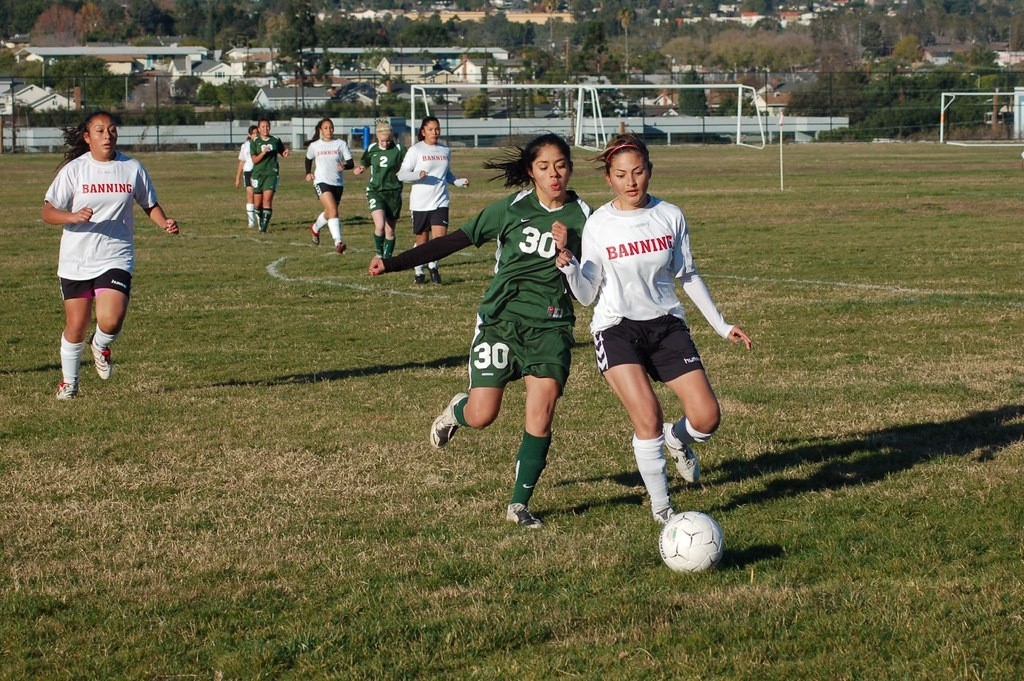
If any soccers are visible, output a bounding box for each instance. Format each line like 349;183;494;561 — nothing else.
659;509;724;575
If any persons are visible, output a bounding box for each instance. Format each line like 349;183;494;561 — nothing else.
234;118;291;236
368;133;596;530
353;119;408;260
555;131;754;528
303;117;355;254
395;116;470;283
41;110;179;401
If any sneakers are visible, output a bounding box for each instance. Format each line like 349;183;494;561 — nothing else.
310;225;319;244
414;271;425;285
430;393;469;449
90;332;112;380
428;266;441;285
653;505;677;526
662;423;700;483
336;242;346;254
506;503;544;530
56;379;79;402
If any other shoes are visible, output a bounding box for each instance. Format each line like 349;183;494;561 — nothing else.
258;226;262;231
259;228;268;236
246;224;256;229
